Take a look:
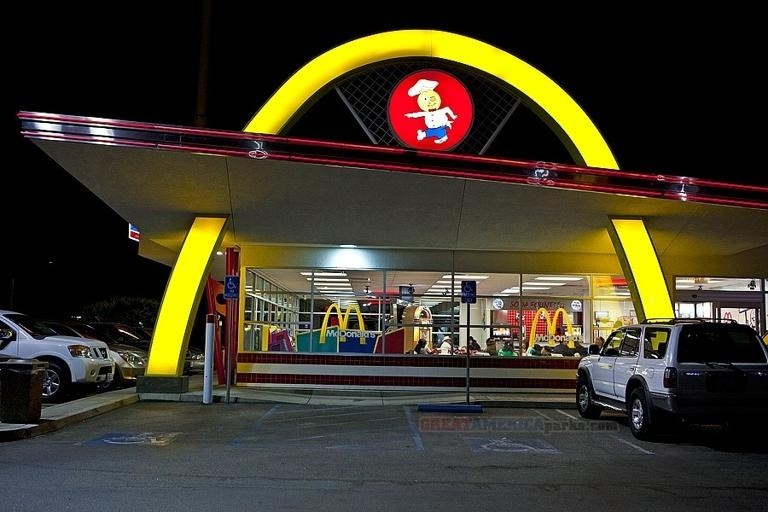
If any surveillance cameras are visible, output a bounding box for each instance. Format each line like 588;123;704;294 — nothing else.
748;281;756;290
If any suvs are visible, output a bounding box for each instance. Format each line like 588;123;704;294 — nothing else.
0;310;116;404
575;314;767;443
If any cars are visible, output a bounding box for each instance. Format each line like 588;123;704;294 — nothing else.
88;321;192;377
134;326;204;376
41;319;147;390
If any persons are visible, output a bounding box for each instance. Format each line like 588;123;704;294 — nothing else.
589;336;605;354
484;327;587;357
468;336;479;350
440;336;458;356
414;339;441;356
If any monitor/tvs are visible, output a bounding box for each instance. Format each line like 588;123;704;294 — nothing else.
399;286;414;300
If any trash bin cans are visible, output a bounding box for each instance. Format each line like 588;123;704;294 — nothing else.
0;358;50;425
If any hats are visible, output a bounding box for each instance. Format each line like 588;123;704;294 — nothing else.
442;335;452;342
